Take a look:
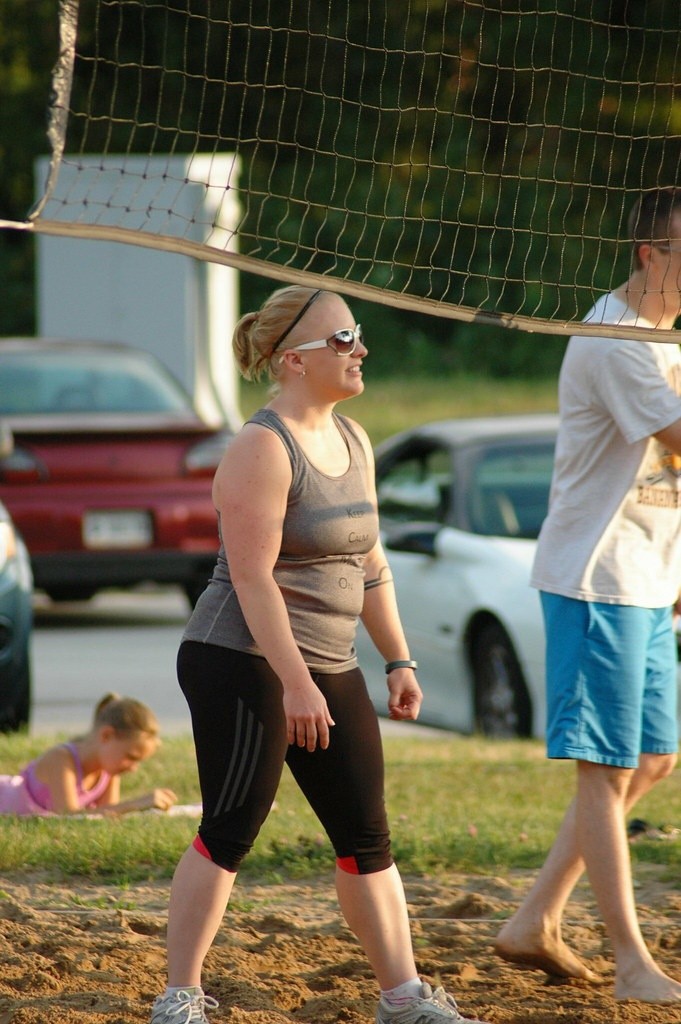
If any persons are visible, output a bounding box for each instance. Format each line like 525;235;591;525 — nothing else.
152;285;479;1024
494;184;681;1005
0;691;179;817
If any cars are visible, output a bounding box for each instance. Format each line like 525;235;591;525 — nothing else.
0;335;233;617
0;503;34;738
354;417;560;743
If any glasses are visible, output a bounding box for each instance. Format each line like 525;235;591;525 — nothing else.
277;324;365;365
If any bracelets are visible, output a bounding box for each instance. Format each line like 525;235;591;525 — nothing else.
385;660;418;675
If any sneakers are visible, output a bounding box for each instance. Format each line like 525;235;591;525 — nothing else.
374;981;491;1024
149;988;220;1024
